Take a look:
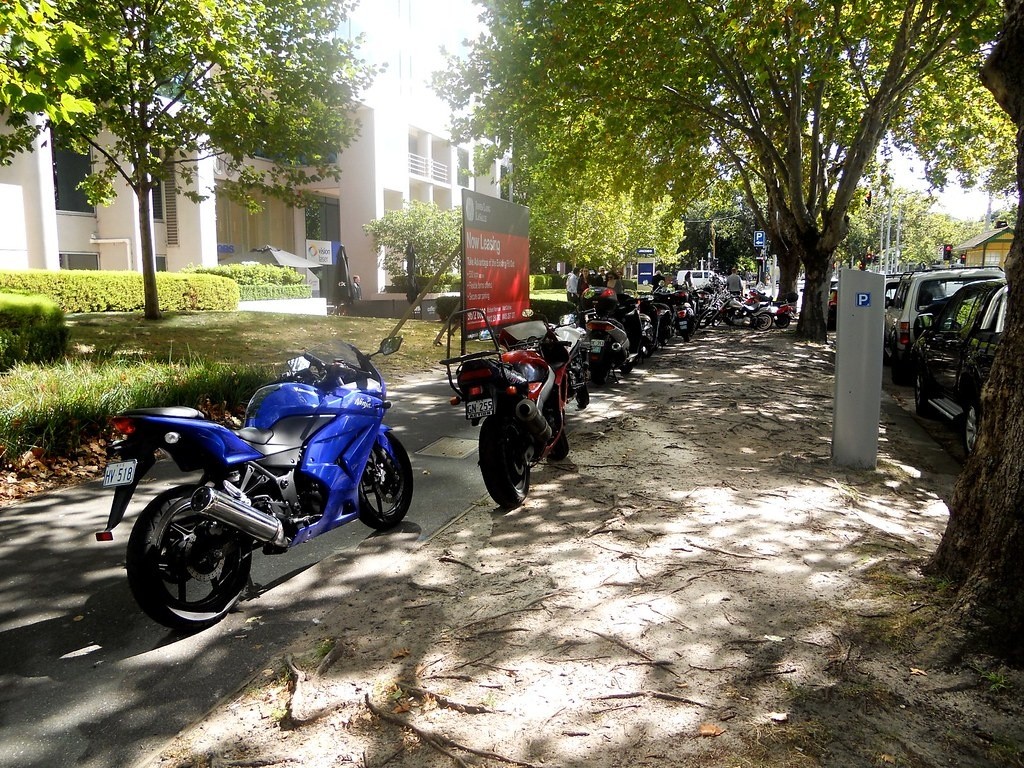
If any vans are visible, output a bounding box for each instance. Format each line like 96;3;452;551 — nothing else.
677;270;724;289
770;267;780;284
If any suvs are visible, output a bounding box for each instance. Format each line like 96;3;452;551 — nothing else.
885;266;1006;386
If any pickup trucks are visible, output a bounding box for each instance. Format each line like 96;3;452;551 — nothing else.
910;276;1009;458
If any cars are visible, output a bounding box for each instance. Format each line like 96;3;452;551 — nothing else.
885;274;907;318
828;282;838;331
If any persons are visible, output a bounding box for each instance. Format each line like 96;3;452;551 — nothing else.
653;271;692;292
727;268;743;297
353;275;361;300
566;266;625;306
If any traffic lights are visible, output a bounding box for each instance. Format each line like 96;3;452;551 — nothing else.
865;191;871;206
961;254;966;264
874;256;879;263
866;254;872;264
943;244;953;260
860;263;865;270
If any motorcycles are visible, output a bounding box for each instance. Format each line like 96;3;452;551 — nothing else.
439;313;576;486
576;276;794;387
95;336;414;633
520;290;589;410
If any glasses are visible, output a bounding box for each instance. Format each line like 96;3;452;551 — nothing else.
607;277;612;278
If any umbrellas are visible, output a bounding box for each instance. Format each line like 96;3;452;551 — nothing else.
335;245;353;306
